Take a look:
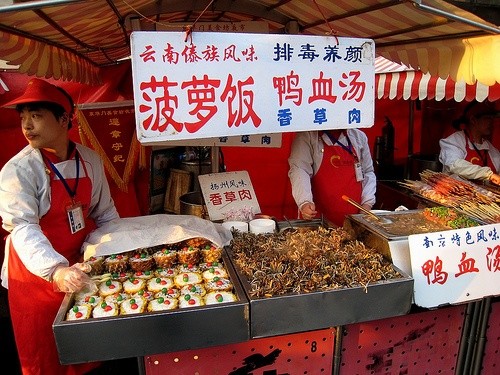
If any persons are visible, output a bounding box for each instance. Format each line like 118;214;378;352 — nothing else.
438;99;500;189
287;127;378;228
76;224;82;230
0;79;123;375
357;164;360;168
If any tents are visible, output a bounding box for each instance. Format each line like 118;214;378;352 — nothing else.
0;1;500;225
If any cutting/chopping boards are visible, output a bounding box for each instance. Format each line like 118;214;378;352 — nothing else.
162;169;191;214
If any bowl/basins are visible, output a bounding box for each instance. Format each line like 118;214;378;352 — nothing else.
249;219;277;235
223;221;248;233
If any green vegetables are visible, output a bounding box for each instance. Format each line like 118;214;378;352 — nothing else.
429;206;481;230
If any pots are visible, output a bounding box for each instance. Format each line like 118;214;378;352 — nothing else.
179;192;207;217
412;151;441;181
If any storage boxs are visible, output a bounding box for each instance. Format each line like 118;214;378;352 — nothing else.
51;217;415;365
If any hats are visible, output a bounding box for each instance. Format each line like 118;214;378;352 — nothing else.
466;101;500;120
0;78;72;114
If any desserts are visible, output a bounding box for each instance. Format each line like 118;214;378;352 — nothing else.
62;236;239;321
